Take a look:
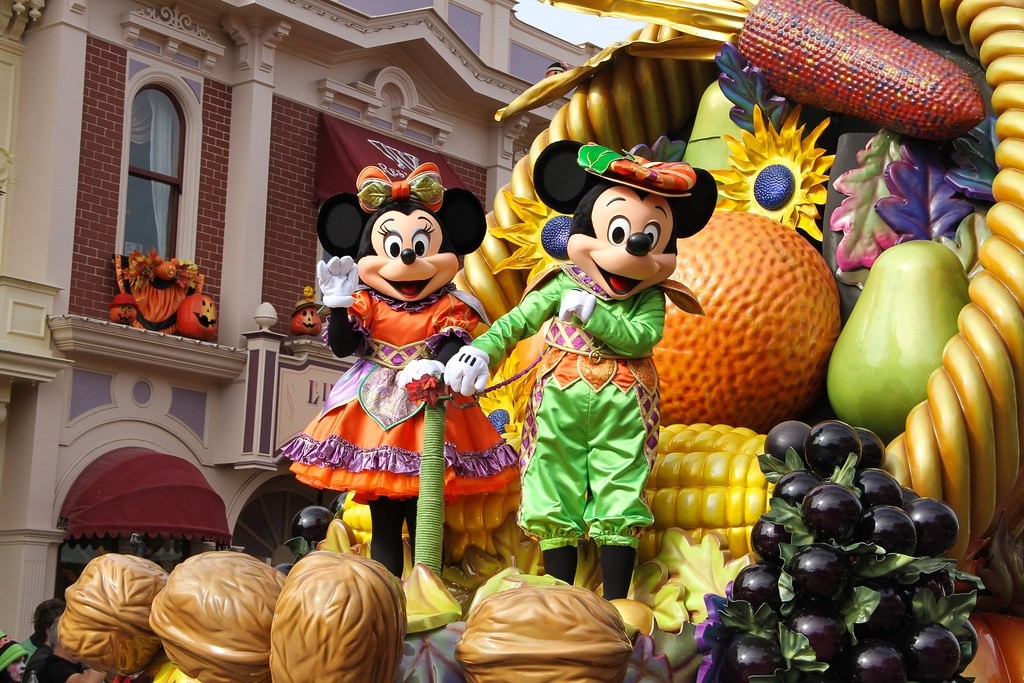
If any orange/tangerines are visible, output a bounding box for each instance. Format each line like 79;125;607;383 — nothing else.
649;210;838;429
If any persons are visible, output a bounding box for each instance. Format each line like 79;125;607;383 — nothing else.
0;630;30;683
22;599;66;683
36;617;107;683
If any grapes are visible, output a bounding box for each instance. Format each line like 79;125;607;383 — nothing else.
290;492;349;546
717;419;979;683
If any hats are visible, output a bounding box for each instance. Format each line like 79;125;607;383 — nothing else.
0;631;29;672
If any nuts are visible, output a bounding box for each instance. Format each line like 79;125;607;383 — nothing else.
55;548;407;683
452;583;633;683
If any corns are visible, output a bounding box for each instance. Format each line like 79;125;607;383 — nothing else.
341;420;776;564
736;0;985;140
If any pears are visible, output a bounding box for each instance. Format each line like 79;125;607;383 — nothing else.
681;77;740;201
825;239;970;436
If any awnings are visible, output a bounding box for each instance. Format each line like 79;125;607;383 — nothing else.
311;113;467;206
61;448;232;551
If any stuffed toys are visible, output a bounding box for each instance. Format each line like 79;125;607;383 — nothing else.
280;162;521;578
444;140;718;601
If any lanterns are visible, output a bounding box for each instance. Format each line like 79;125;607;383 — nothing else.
177;294;218;341
290;300;322;337
110;294;140;325
